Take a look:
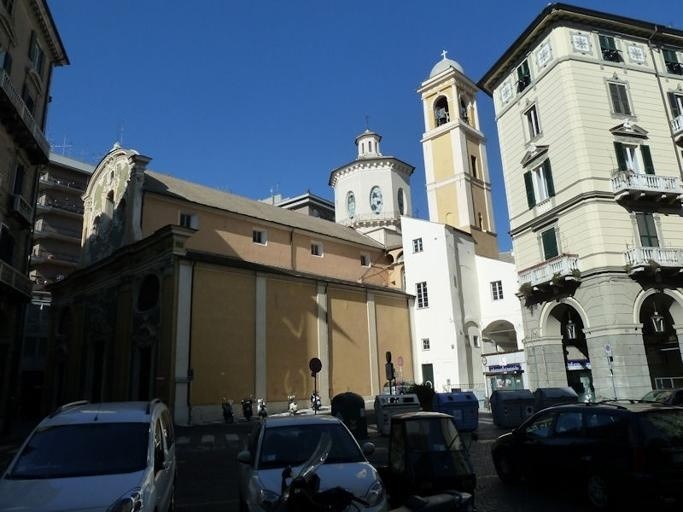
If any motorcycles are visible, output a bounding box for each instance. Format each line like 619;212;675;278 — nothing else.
286;393;299;418
256;397;269;422
220;396;235;425
309;391;323;412
383;411;481;512
249;433;370;511
240;398;254;422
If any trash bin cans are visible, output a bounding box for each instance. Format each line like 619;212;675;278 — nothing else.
490;387;579;429
374;393;421;437
331;392;367;439
432;392;479;432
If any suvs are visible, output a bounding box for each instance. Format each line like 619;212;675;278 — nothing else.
1;398;182;512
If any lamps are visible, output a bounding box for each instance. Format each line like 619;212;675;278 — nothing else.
648;302;664;333
564;311;576;339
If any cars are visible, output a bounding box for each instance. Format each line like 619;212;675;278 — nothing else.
488;396;683;512
235;410;391;512
383;381;415;397
638;387;683;410
